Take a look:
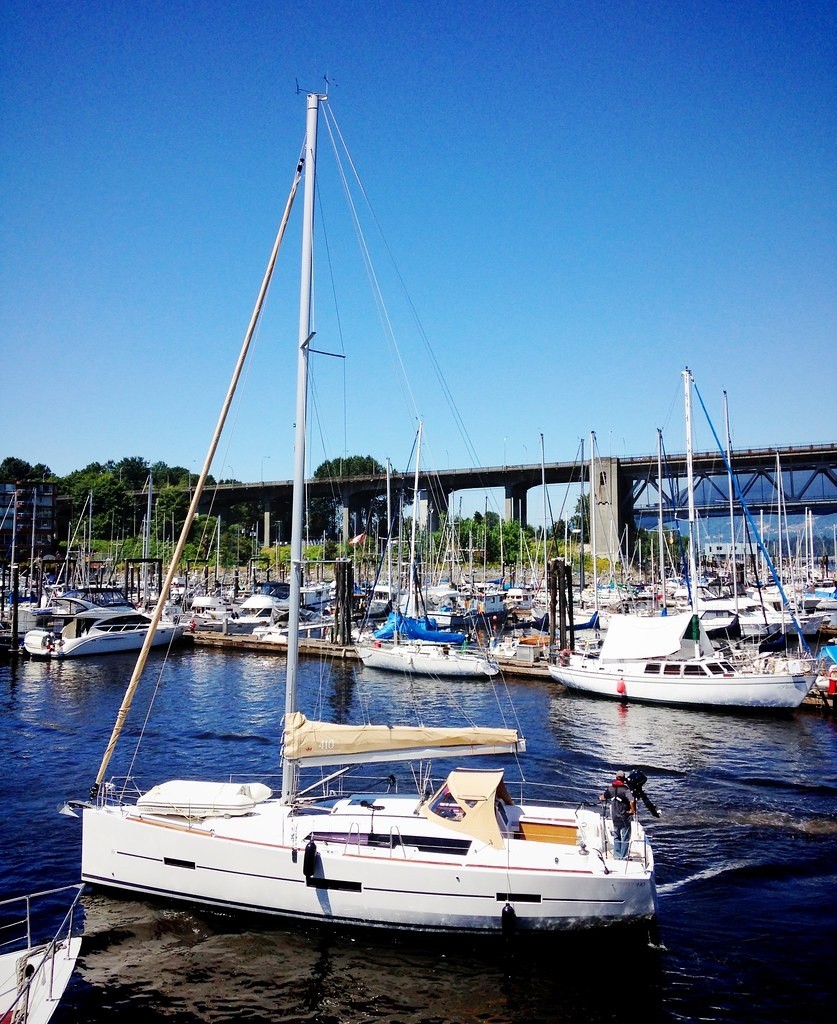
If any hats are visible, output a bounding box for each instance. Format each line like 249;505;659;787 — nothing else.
616;771;625;779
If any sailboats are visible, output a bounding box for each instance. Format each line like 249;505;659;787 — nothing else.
0;365;837;717
58;71;661;940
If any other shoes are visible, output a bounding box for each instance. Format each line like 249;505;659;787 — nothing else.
622;856;633;861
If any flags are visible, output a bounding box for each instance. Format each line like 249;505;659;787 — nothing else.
347;532;365;544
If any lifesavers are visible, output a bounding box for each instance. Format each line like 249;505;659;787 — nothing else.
558;648;573;667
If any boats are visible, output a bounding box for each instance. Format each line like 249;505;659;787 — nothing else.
0;883;89;1024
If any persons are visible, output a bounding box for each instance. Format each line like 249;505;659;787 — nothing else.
597;770;635;861
